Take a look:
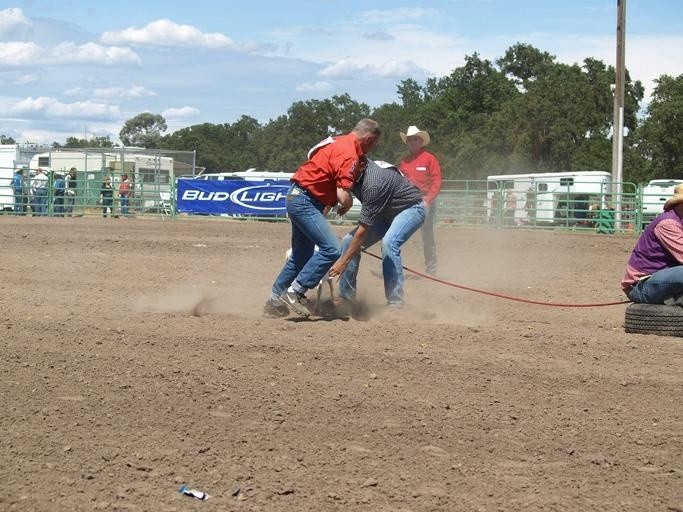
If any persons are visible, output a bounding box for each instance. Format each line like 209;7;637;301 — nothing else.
98;176;113;218
620;183;682;309
393;122;439;275
308;138;425;318
261;118;381;321
116;173;132;214
472;176;538;225
114;175;130;198
7;166;77;219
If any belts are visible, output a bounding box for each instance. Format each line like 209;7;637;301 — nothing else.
622;283;636;292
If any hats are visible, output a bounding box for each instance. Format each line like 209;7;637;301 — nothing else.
663;183;683;210
398;125;431;147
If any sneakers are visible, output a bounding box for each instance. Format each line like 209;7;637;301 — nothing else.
323;296;353;307
269;291;308;307
278;288;310;317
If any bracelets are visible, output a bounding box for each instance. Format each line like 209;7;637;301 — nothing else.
99;198;102;199
